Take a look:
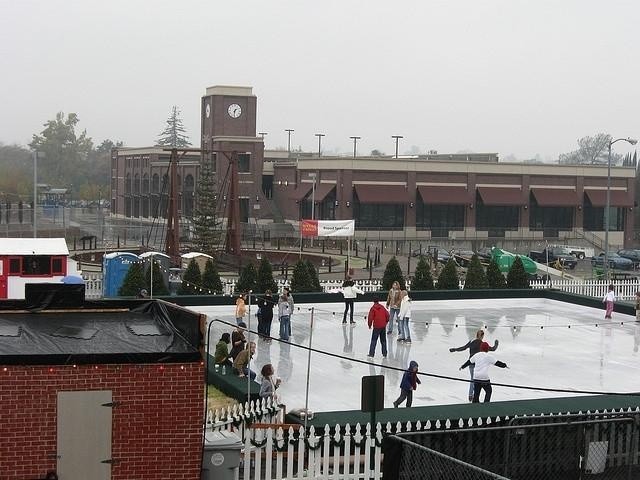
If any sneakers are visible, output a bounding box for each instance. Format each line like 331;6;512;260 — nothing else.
350;321;356;324
404;339;412;342
387;331;392;334
342;319;348;323
367;354;374;357
397;338;405;342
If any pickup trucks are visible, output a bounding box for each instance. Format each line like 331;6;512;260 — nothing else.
528;246;580;269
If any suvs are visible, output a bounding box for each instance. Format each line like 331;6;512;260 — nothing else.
617;248;640;267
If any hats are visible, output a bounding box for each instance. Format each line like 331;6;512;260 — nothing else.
480;342;490;350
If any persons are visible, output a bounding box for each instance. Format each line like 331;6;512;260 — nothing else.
602;284;618;319
633;292;640;323
459;341;508;404
385;280;402;335
258;363;281;406
396;290;413;343
255;336;294;383
340;323;356;369
338;275;365;326
367;296;392;359
449;329;500;402
393;360;421;408
368;335;411;388
213;286;294;381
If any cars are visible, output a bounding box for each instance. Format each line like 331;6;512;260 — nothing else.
415;243;511;270
51;197;111;209
591;251;634;270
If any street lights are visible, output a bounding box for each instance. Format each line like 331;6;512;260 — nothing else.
97;184;102;209
604;138;639;281
286;127;296;156
307;172;317;246
391;135;405;160
69;183;75;207
31;150;46;239
259;130;267;150
349;135;362;156
315;133;327;160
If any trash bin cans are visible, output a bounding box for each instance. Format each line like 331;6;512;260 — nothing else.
200;430;245;480
